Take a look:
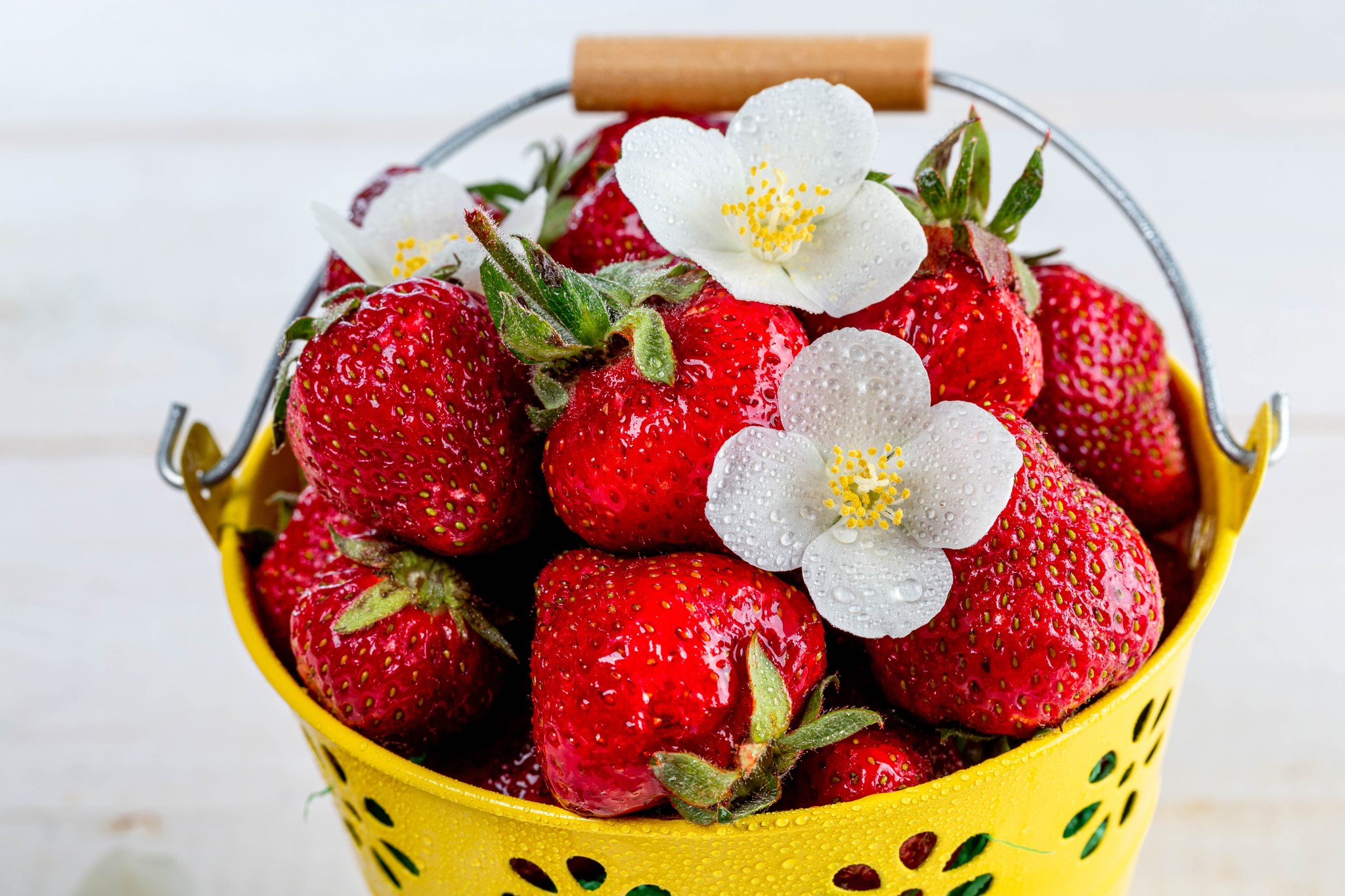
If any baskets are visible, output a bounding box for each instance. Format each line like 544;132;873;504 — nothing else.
155;37;1291;896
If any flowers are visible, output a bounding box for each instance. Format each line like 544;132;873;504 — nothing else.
703;328;1026;640
614;76;929;319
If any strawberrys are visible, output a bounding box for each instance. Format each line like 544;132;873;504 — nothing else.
242;102;1195;891
